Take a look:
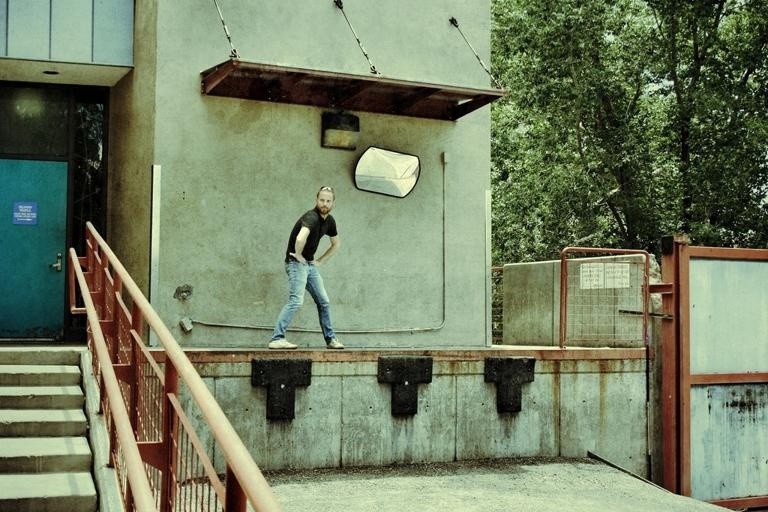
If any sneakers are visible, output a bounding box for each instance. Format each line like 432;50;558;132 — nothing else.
268;336;299;347
325;336;345;349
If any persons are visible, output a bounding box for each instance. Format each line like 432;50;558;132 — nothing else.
268;185;347;349
